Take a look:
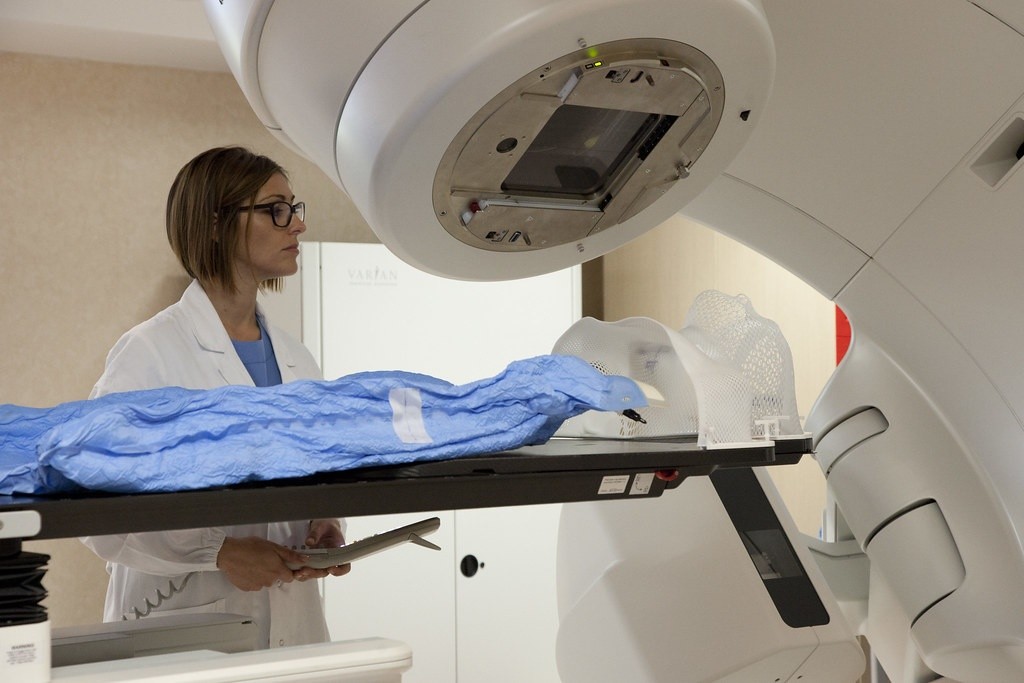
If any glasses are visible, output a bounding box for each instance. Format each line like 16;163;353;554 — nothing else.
239;201;306;227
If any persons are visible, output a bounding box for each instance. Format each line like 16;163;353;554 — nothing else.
78;144;351;645
